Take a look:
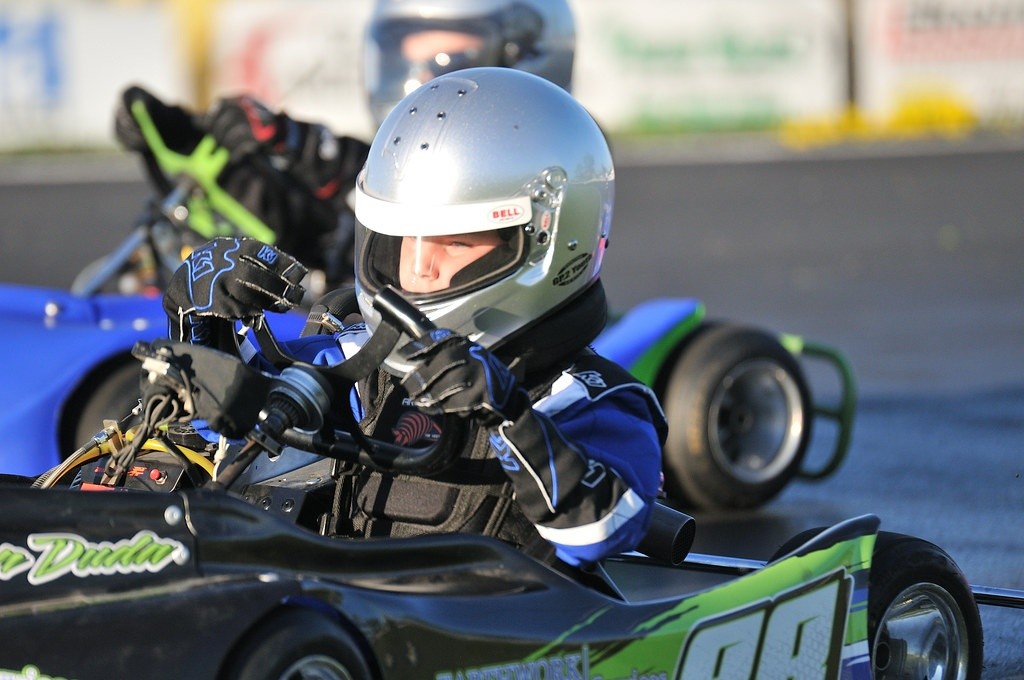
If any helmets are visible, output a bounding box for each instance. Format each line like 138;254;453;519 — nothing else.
362;0;575;132
353;67;615;372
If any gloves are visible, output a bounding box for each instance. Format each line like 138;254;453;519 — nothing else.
162;237;308;366
117;105;192;151
397;328;588;523
203;96;300;172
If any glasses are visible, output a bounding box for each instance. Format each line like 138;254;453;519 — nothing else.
379;52;483;80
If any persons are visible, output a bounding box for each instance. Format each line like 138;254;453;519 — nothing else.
114;0;574;287
159;62;670;588
